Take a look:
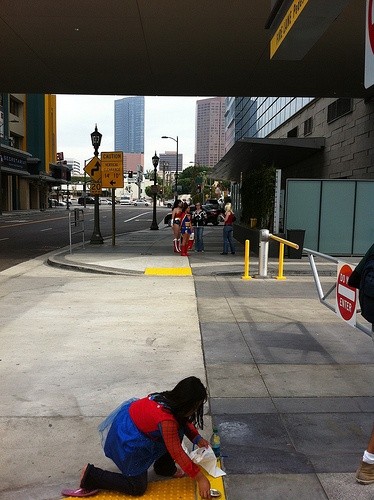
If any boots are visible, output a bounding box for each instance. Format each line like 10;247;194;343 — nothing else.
177;239;182;252
188;240;195;251
173;239;180;254
181;245;189;256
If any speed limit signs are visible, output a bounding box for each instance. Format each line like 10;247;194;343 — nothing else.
90;183;102;195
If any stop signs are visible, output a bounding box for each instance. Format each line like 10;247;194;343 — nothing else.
334;260;357;327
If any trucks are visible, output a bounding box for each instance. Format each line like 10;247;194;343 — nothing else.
163;194;193;208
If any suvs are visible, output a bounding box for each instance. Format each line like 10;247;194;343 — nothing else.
204;199;220;210
78;197;95;206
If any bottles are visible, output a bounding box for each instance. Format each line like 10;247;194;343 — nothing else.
210;429;221;459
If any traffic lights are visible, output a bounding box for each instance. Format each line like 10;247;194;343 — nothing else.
56;152;63;161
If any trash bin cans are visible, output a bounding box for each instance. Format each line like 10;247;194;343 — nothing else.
287;229;306;259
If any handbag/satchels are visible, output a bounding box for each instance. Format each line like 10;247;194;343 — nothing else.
232;216;236;222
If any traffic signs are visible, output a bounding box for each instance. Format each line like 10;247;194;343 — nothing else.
100;152;124;187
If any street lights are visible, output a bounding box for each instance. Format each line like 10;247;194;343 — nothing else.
150;151;160;230
161;135;178;203
189;161;201;193
90;123;105;244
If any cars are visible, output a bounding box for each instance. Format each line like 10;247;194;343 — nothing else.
163;204;221;227
97;196;120;205
50;195;79;206
132;199;151;207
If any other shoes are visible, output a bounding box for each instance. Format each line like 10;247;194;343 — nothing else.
194;249;200;252
202;249;205;253
220;253;228;255
355;461;374;484
231;252;235;254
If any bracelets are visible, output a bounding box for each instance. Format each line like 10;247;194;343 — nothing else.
193;435;202;452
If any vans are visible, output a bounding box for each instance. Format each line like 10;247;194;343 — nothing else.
120;195;132;205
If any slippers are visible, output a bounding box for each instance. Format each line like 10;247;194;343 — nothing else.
61;487;98;496
154;468;185;480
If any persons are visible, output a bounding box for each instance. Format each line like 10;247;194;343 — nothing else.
66;197;72;208
171;199;207;256
355;426;374;485
219;202;236;255
79;376;212;498
